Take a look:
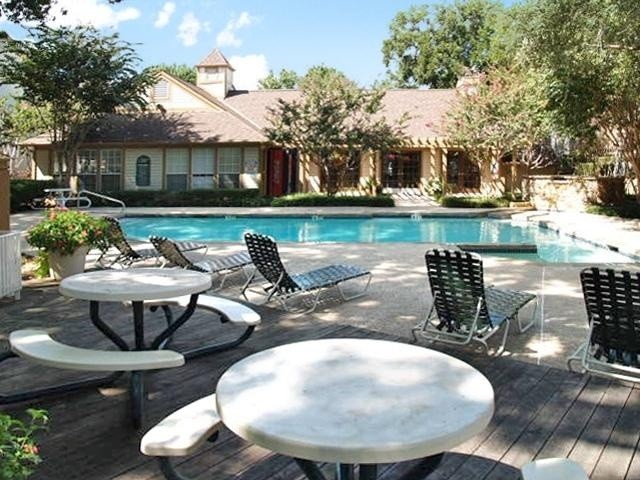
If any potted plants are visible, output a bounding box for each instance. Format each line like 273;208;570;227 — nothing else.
25;205;111;282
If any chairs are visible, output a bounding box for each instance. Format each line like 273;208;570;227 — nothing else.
95;216;209;267
566;267;640;383
149;235;253;293
239;233;372;315
411;249;539;358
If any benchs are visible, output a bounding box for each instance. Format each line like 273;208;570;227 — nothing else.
521;457;589;480
8;328;186;430
143;293;263;360
140;392;223;479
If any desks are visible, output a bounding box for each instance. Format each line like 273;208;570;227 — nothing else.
214;338;495;479
58;266;212;351
